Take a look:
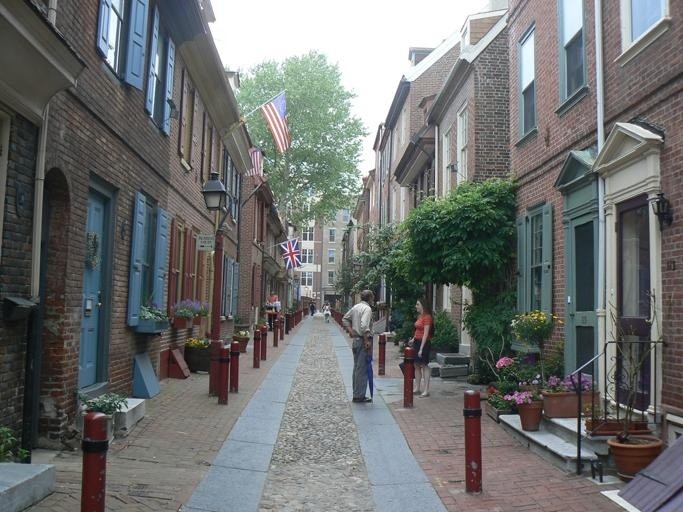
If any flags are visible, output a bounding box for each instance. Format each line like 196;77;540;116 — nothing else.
242;149;264;178
262;90;291;154
280;240;304;270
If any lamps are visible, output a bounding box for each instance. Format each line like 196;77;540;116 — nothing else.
651;190;674;232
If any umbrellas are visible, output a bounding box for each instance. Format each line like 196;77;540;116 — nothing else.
365;342;376;400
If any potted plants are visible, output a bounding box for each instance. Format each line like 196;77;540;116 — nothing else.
73;386;129;444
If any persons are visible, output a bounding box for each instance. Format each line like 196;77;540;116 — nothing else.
265;291;278;330
321;303;331;322
407;295;434;398
341;290;379;404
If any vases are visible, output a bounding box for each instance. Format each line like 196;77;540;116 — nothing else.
191;315;201;325
182;343;213;371
170;317;188;329
134;319;168;334
231;335;250;353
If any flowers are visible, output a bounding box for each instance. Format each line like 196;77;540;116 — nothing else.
173;298;199;320
183;335;212;350
482;307;667;483
234;328;251;337
138;298;168;321
192;296;209;317
